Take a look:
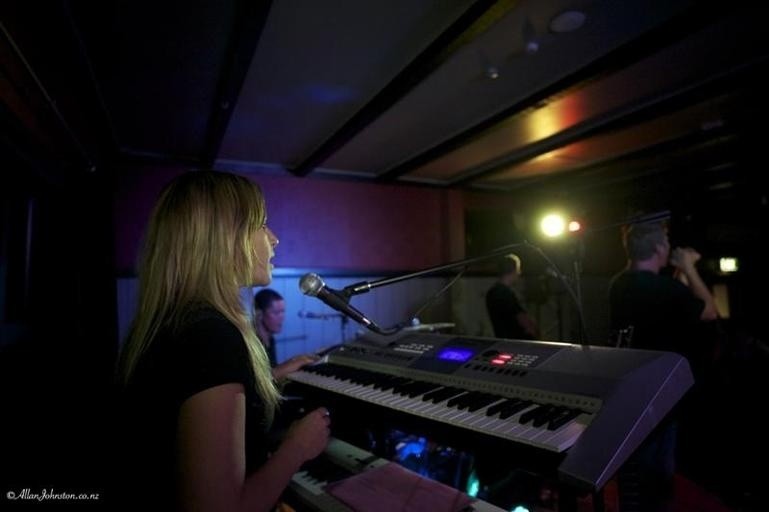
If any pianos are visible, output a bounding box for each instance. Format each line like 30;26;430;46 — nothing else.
266;330;694;512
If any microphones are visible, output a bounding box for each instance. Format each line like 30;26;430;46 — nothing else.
299;273;371;328
297;310;343;321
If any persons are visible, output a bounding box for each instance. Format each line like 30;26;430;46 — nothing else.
485;253;540;340
253;289;285;368
99;172;329;509
610;222;718;353
